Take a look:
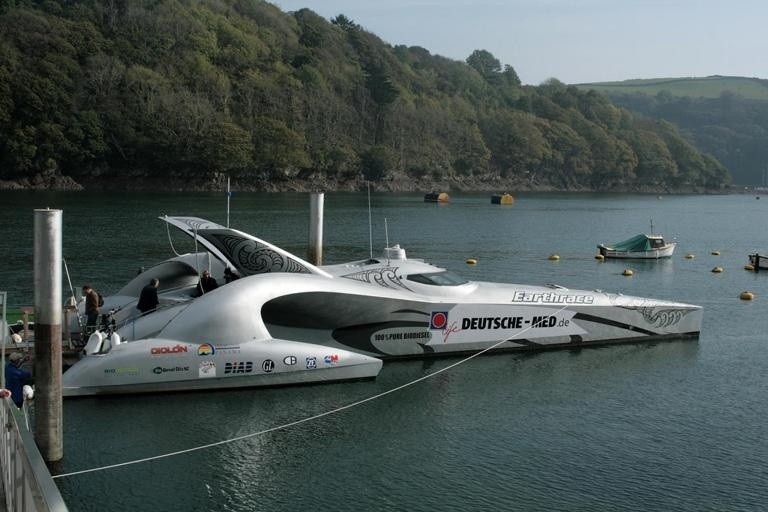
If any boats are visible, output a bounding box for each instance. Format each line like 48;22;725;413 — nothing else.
1;217;702;396
598;234;677;259
748;253;768;269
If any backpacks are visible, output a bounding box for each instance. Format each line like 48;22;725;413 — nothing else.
97;292;104;307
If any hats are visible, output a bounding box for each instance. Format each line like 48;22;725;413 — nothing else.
9;352;22;360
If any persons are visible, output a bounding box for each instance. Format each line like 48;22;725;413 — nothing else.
82;286;98;333
222;266;240;283
0;389;12;399
134;279;160;317
195;269;219;294
5;352;33;409
